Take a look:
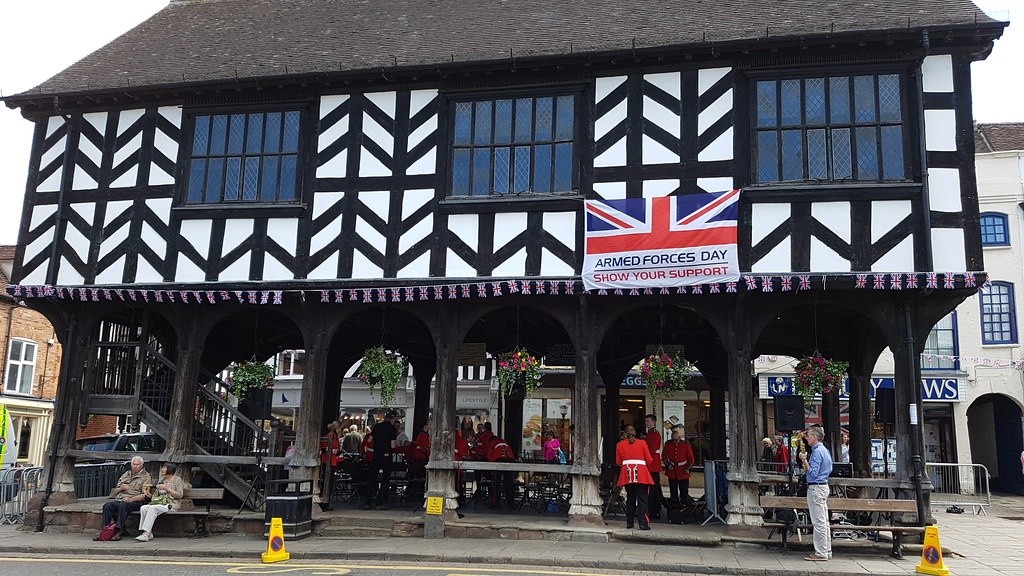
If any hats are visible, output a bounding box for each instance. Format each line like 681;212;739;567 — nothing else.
478;424;483;428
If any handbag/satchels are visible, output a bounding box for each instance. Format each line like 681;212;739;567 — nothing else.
557;447;566;463
100;521;117;541
150;491;170;505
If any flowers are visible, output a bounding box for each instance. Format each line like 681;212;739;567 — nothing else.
641;347;698;399
357;345;408;407
230;359;276;399
791;352;849;402
496;347;543;399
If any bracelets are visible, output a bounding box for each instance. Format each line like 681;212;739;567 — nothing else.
802;459;806;463
169;488;172;493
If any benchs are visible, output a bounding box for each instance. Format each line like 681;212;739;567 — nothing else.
103;487;225;536
757;496;927;560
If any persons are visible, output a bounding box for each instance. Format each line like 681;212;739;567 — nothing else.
568;424;574;463
798;423;833;561
365;413;395;510
616;425;651;529
487;436;515;509
841;433;852;462
93;456;152;540
662;426;694;504
761;432;832;475
644;414;663;520
135;462;183;542
544;431;560;465
454;422;493;505
319;419;432;510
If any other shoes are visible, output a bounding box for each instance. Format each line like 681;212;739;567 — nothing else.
111;532;122;541
804;554;828;560
135;533;153;542
639;525;650;530
364;505;369;509
93;535;100;541
376;506;386;510
627;524;634;528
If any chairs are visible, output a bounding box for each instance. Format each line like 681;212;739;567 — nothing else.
331;449;632;517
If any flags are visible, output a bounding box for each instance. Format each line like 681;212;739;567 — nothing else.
320;280;590;303
581;189;740;290
598;276;810;294
855;272;977;289
14;284;283;304
927;355;1022;369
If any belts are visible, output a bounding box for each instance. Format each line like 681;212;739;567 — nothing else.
326;447;335;453
495;443;508;448
367;448;374;452
655;450;660;453
417;446;421;449
670;461;687;466
622;460;646;465
808;483;826;485
455;449;458;453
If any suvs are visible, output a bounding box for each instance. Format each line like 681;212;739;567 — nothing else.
74;432;165;494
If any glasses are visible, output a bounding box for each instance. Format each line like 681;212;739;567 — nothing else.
344;432;348;433
672;439;678;442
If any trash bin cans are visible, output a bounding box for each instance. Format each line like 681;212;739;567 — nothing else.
264;479;315;541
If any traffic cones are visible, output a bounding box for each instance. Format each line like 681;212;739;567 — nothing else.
915;525;950;576
260;518;290;563
